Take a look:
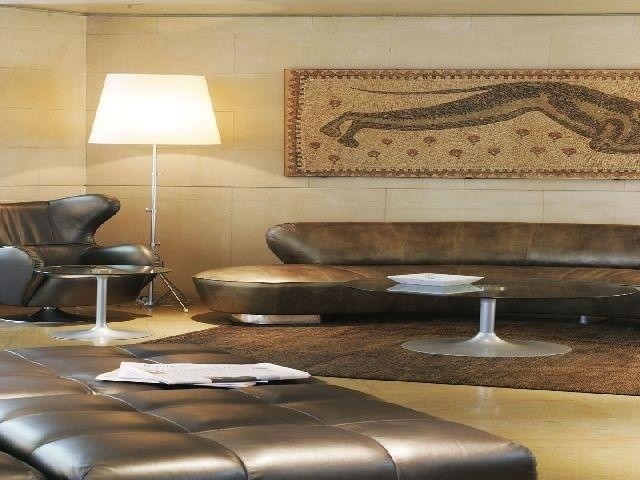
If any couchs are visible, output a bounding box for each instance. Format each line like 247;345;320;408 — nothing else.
1;195;156;324
191;222;639;325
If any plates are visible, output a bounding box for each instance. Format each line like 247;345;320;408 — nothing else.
387;271;485;286
386;286;486;295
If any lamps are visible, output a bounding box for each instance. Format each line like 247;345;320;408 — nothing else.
87;72;221;309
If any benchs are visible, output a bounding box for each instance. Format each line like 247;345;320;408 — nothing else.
1;343;536;479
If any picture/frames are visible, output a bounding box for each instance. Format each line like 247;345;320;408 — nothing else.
282;65;639;180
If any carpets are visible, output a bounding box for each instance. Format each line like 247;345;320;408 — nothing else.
151;321;640;397
